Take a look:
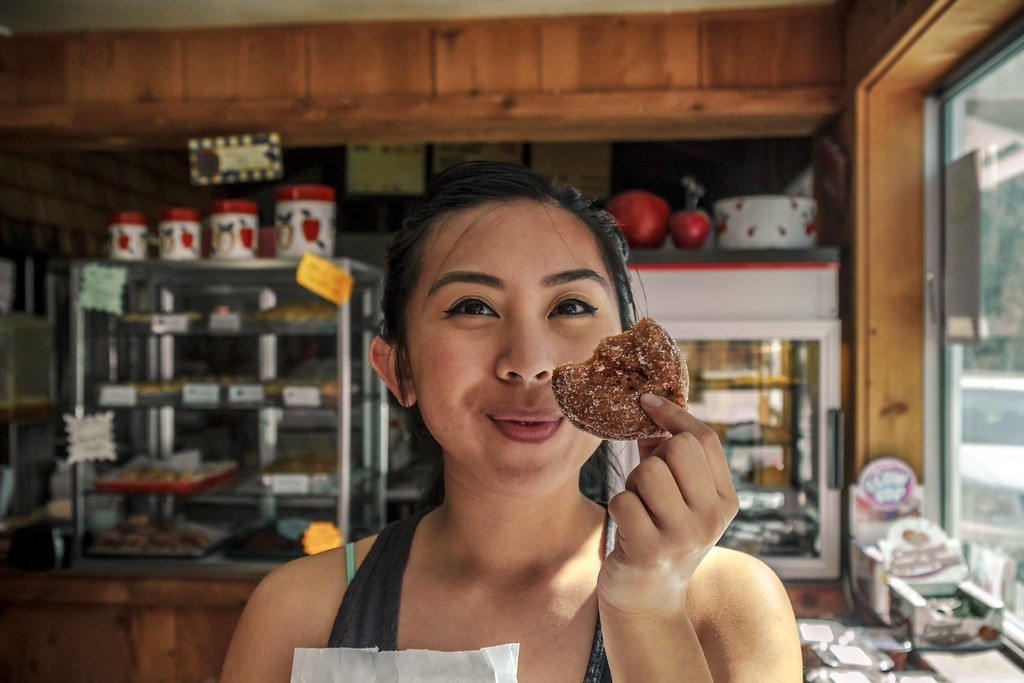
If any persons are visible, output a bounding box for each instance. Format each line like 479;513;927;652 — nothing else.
217;156;805;683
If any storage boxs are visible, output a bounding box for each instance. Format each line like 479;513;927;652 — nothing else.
886;574;1006;651
851;542;894;626
847;485;927;539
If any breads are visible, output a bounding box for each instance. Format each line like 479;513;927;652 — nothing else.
549;317;689;441
92;301;338;553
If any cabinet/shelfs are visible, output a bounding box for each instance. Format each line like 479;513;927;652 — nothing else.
68;254;390;581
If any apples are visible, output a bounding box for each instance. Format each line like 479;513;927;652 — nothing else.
668;208;711;251
603;188;669;249
